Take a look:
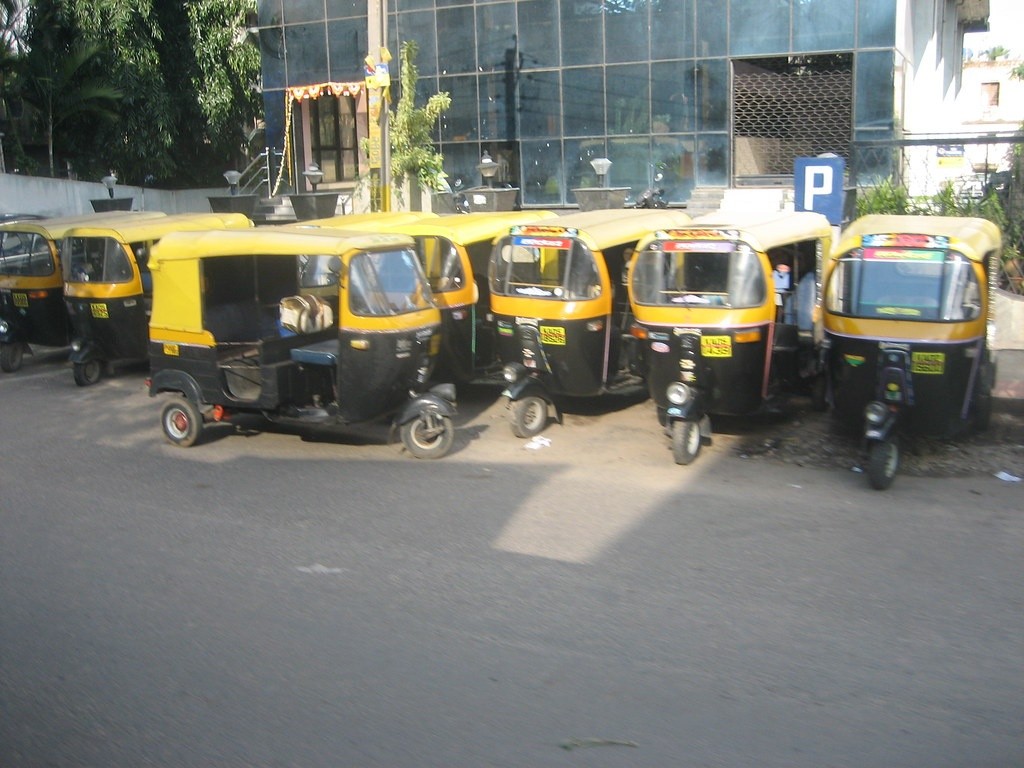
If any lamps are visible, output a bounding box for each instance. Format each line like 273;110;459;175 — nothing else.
101;176;118;197
219;171;244;195
587;157;613;187
476;150;498;187
303;160;324;193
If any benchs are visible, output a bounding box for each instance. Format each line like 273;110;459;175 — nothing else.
784;272;814;326
207;303;275;362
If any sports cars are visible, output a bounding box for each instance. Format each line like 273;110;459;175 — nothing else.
143;225;460;461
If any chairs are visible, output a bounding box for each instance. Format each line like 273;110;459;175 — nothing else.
275;292;341;367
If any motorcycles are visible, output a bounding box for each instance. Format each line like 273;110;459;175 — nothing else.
0;211;164;374
288;209;566;388
60;211;258;385
624;208;835;466
817;213;1002;493
483;208;686;444
